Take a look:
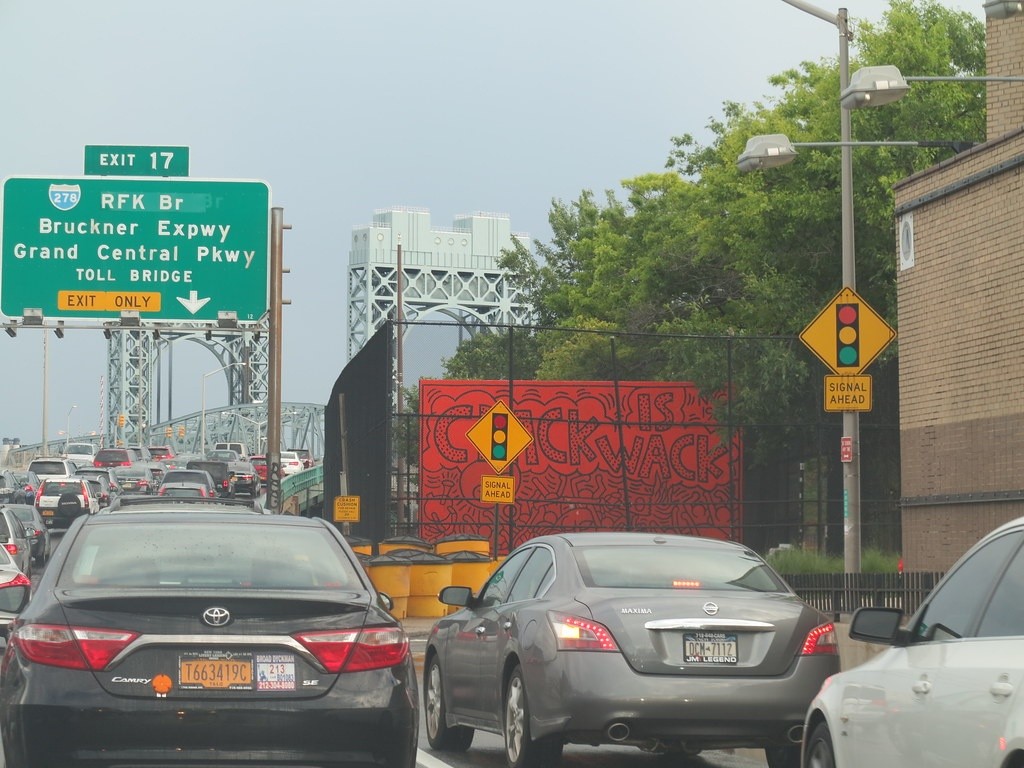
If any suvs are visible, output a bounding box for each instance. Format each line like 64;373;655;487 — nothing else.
147;445;176;460
93;448;138;467
0;469;27;504
27;457;78;482
74;467;125;496
34;478;103;528
67;474;118;510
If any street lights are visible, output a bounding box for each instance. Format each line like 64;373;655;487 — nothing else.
66;405;77;446
202;363;247;460
221;412;298;455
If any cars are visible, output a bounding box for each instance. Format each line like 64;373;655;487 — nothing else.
147;454;209;481
0;543;31;644
228;461;261;497
248;455;287;484
115;466;154;495
287;448;315;469
59;444;100;463
11;471;41;505
801;517;1024;767
0;505;36;580
422;532;841;768
0;504;54;567
157;483;210;505
206;450;239;463
118;446;152;462
159;469;223;505
214;442;256;461
187;461;235;505
280;451;304;475
0;514;421;767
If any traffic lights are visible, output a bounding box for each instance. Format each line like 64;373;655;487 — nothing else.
166;428;171;438
491;412;507;461
836;303;860;368
119;415;124;428
179;426;185;437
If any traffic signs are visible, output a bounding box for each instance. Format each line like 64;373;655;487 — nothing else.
2;174;271;326
